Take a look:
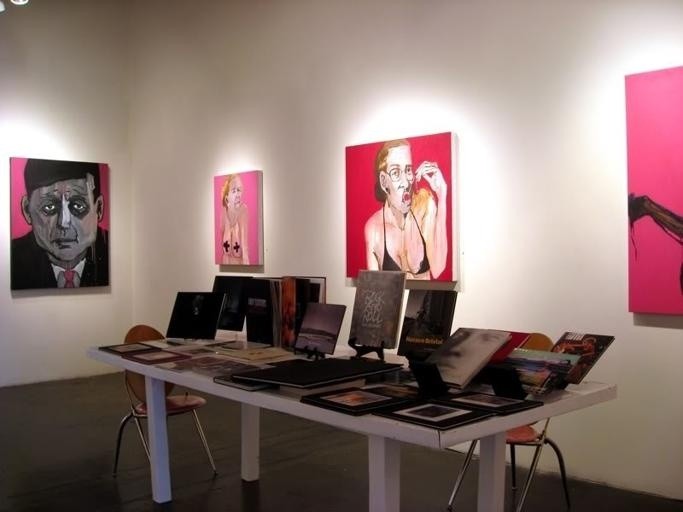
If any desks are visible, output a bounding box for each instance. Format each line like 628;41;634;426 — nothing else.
87;335;616;512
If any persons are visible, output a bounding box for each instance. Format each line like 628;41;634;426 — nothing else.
11;158;107;291
218;175;249;264
363;140;449;281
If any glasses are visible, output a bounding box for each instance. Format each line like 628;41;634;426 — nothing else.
384;166;414;182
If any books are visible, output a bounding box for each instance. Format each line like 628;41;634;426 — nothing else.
96;270;614;431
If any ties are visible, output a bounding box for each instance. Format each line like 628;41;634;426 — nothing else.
62;270;75;288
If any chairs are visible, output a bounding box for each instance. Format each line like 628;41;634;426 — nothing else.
112;325;218;474
446;417;570;512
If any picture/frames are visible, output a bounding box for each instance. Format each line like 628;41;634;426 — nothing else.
122;349;192;364
429;391;543;415
99;342;162;355
370;397;496;429
299;387;411;416
